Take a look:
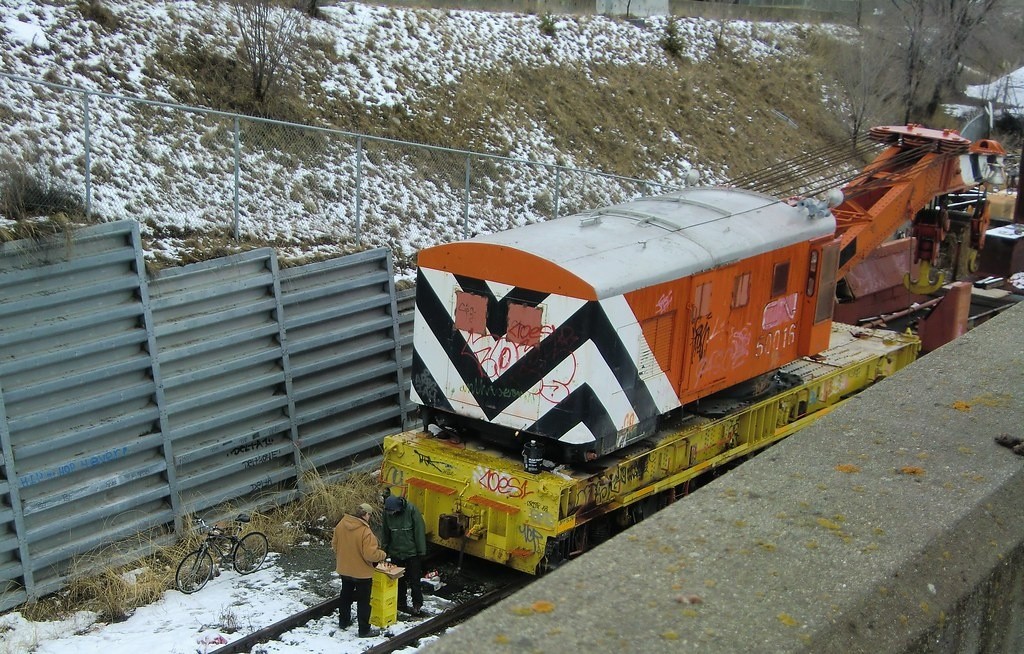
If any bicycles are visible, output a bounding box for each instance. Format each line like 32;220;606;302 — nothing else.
176;512;269;594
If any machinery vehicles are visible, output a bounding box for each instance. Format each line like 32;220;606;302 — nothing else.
379;120;1004;581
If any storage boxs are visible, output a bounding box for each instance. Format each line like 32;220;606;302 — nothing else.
368;571;398;629
988;195;1016;221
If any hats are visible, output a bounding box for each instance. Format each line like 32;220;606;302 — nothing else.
386;495;401;511
361;504;374;514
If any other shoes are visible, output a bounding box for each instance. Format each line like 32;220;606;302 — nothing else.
398;604;406;609
361;630;381;637
414;605;421;614
341;622;353;629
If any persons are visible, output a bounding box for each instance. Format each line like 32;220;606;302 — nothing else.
331;503;387;638
381;494;426;614
